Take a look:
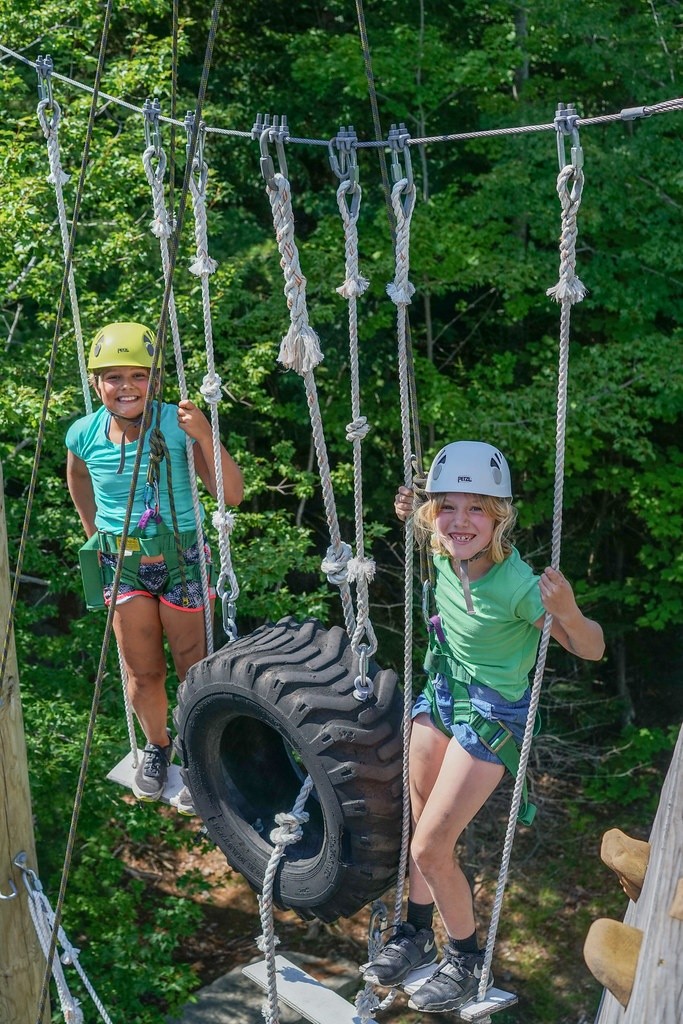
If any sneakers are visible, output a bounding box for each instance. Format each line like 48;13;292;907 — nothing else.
362;921;438;988
407;944;495;1012
177;785;197;816
131;734;175;803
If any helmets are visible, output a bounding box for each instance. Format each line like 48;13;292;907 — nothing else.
87;323;162;369
424;441;512;498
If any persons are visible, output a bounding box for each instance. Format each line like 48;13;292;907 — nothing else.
65;322;244;815
363;440;605;1013
